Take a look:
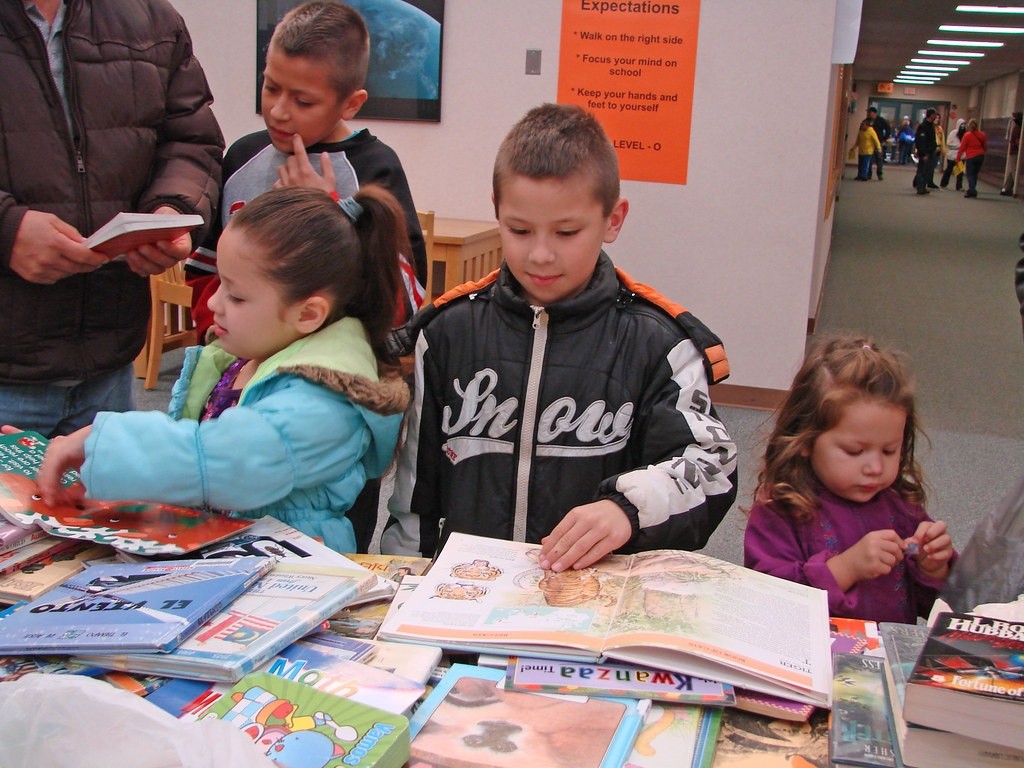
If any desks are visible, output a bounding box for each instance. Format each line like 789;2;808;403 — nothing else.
133;218;503;380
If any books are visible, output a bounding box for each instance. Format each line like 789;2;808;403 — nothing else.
82;212;206;261
377;531;833;768
0;432;434;768
902;609;1024;768
825;616;932;768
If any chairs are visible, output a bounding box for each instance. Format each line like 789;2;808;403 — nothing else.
416;211;435;310
144;259;194;390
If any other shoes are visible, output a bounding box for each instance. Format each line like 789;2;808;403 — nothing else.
916;185;930;195
877;174;884;181
940;184;948;188
867;175;872;179
854;176;868;181
928;183;939;189
964;191;979;198
956;185;963;191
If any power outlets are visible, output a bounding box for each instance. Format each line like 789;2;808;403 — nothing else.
525;49;541;75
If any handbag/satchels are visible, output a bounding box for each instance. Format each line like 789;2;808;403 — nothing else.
905;134;917;145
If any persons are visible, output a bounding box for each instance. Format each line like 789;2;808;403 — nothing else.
184;1;427;354
941;117;989;198
912;108;945;195
896;117;920;164
32;187;409;557
850;108;891;181
0;0;222;441
744;333;957;622
381;104;739;573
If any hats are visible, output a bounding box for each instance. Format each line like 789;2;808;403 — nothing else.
865;107;877;113
904;115;909;119
